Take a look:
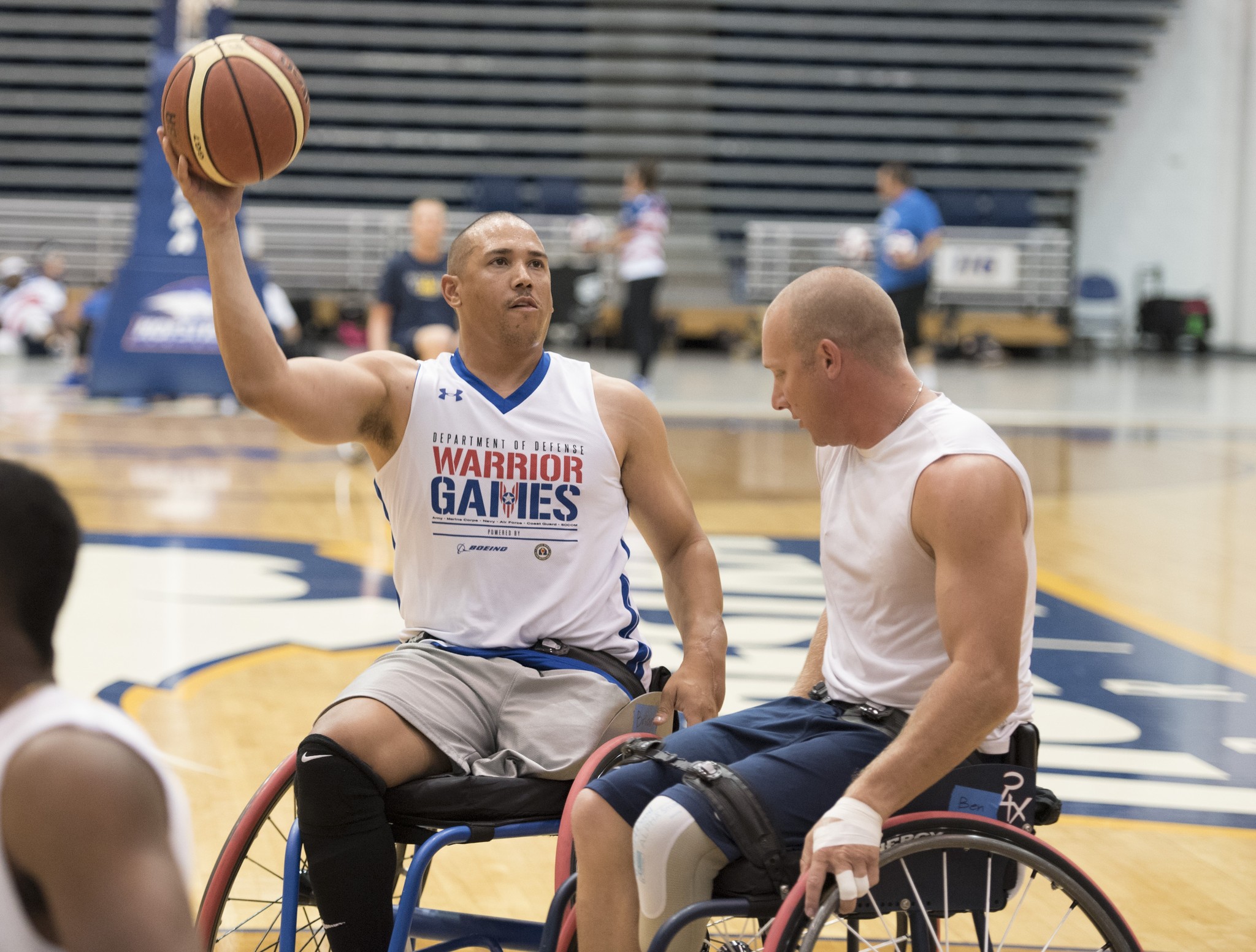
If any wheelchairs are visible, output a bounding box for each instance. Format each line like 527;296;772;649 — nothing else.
536;719;1145;952
193;665;665;951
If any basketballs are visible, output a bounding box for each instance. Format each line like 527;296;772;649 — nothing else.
162;34;311;187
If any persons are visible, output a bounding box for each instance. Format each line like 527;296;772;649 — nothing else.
0;256;109;388
158;125;728;952
852;159;943;348
0;460;197;952
570;266;1037;952
602;158;669;390
366;198;459;361
